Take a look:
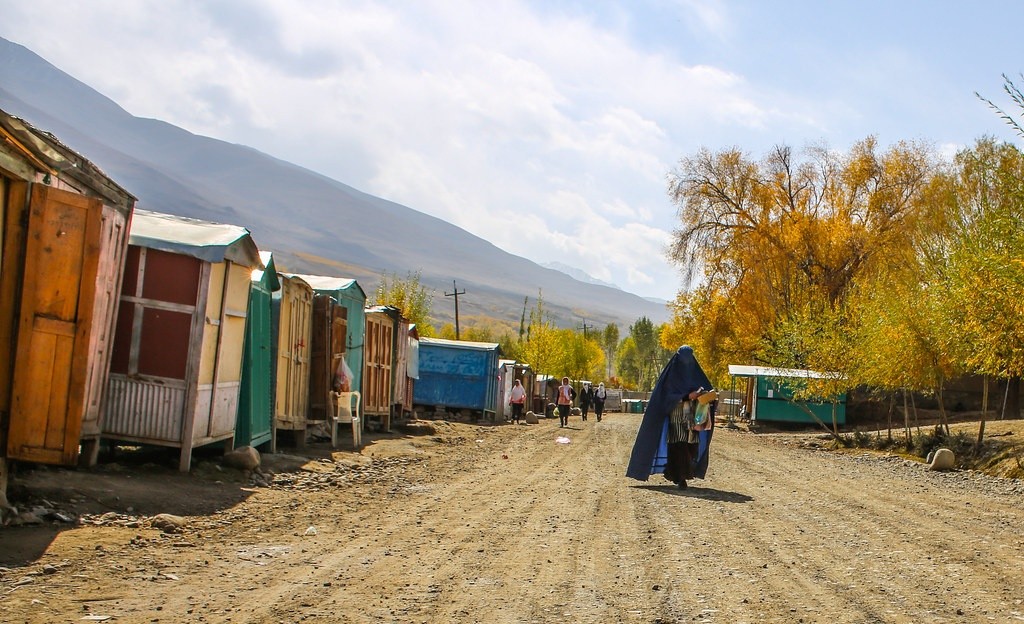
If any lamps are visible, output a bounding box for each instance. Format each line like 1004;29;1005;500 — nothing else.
43;172;52;184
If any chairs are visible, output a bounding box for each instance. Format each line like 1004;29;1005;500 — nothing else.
331;391;363;448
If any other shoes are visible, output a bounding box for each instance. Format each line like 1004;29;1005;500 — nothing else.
511;420;514;424
564;421;568;425
517;420;520;424
679;480;687;489
560;424;563;428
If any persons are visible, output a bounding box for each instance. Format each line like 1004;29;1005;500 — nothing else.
665;346;717;491
579;383;593;421
506;379;526;425
555;377;577;428
595;382;607;420
